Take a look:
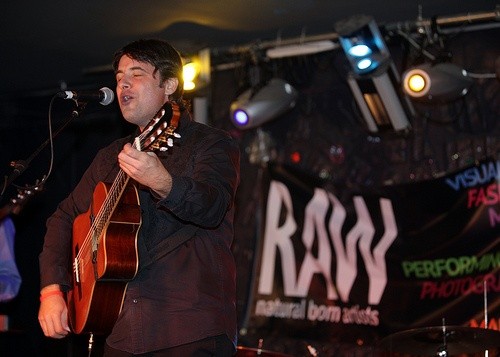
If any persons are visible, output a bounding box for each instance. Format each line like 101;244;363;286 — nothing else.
39;39;240;357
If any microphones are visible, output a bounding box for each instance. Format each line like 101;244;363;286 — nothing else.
58;87;114;106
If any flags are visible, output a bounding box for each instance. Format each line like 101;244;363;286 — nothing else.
244;158;500;335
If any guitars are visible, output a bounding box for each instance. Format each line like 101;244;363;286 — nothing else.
64;100;181;338
0;176;46;221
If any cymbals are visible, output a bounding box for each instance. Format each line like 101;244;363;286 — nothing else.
235;345;294;357
379;325;500;357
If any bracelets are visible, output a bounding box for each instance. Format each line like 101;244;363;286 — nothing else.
39;290;65;301
